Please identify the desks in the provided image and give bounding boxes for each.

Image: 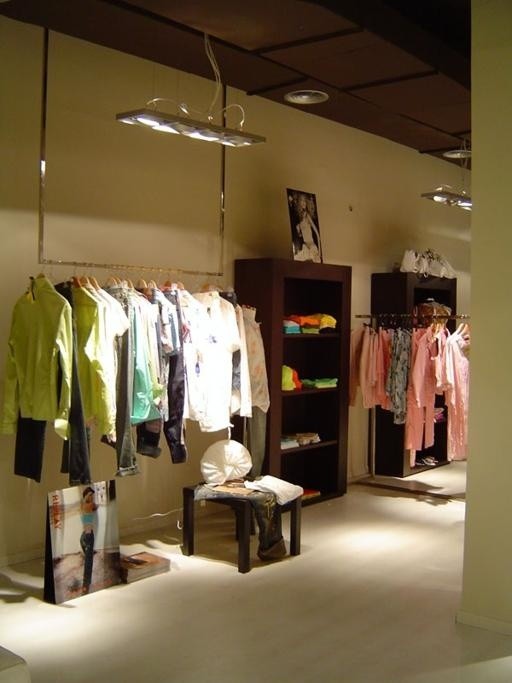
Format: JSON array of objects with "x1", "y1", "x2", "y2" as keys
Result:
[{"x1": 182, "y1": 478, "x2": 302, "y2": 572}]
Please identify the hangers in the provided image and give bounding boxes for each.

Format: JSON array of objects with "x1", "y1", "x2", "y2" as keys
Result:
[
  {"x1": 41, "y1": 261, "x2": 222, "y2": 294},
  {"x1": 366, "y1": 315, "x2": 464, "y2": 332}
]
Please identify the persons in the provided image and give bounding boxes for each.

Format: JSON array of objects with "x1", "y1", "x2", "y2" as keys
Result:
[
  {"x1": 79, "y1": 487, "x2": 99, "y2": 597},
  {"x1": 293, "y1": 194, "x2": 322, "y2": 264}
]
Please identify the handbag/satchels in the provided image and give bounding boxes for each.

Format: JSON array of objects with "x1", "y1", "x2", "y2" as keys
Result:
[
  {"x1": 400, "y1": 250, "x2": 430, "y2": 277},
  {"x1": 425, "y1": 250, "x2": 446, "y2": 278},
  {"x1": 439, "y1": 255, "x2": 457, "y2": 279}
]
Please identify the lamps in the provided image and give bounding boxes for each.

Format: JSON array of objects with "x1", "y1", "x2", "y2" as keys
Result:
[
  {"x1": 421, "y1": 140, "x2": 472, "y2": 212},
  {"x1": 117, "y1": 36, "x2": 266, "y2": 148}
]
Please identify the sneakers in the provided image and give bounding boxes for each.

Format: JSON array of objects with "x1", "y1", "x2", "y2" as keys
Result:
[{"x1": 415, "y1": 456, "x2": 439, "y2": 467}]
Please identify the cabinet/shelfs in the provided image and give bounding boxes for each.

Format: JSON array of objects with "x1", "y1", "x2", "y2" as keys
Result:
[
  {"x1": 230, "y1": 259, "x2": 351, "y2": 512},
  {"x1": 369, "y1": 273, "x2": 457, "y2": 478}
]
